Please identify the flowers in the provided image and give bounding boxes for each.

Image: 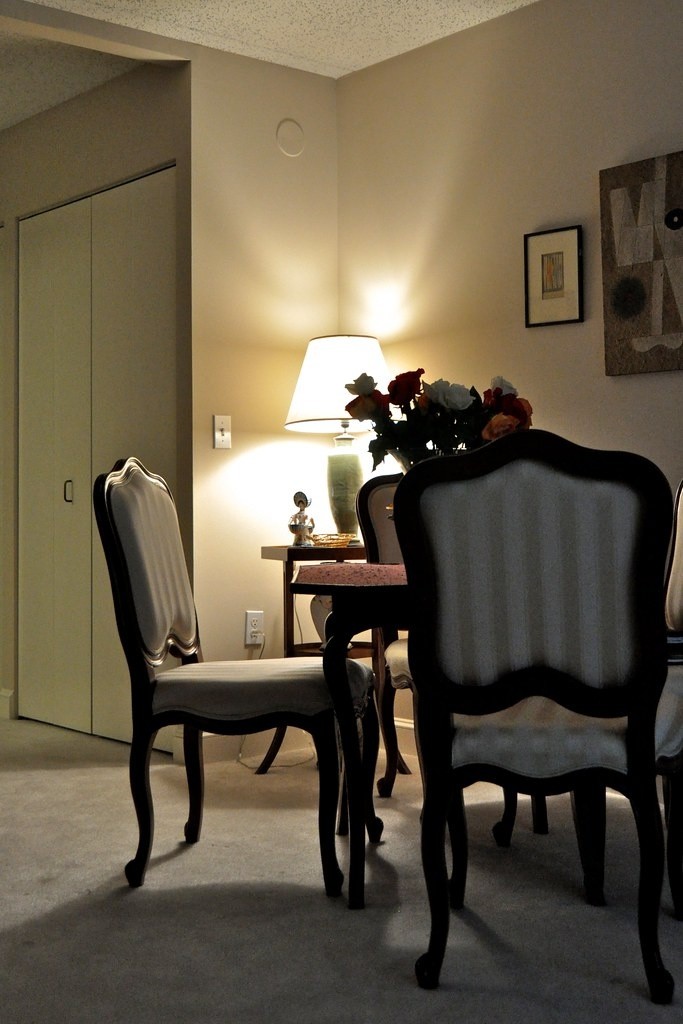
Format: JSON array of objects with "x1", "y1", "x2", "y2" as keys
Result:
[{"x1": 342, "y1": 368, "x2": 533, "y2": 473}]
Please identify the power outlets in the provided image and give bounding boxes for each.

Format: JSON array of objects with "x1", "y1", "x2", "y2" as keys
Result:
[{"x1": 246, "y1": 609, "x2": 266, "y2": 645}]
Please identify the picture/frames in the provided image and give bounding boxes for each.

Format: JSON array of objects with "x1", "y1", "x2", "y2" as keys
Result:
[{"x1": 524, "y1": 225, "x2": 584, "y2": 327}]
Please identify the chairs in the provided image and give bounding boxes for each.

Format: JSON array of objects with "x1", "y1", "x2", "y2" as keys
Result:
[
  {"x1": 95, "y1": 458, "x2": 384, "y2": 895},
  {"x1": 353, "y1": 473, "x2": 409, "y2": 795},
  {"x1": 393, "y1": 429, "x2": 676, "y2": 1006}
]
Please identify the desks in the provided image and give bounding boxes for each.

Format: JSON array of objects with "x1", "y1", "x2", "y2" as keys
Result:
[
  {"x1": 287, "y1": 561, "x2": 681, "y2": 909},
  {"x1": 253, "y1": 546, "x2": 413, "y2": 776}
]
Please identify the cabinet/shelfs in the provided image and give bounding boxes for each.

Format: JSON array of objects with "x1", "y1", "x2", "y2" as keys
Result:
[{"x1": 18, "y1": 161, "x2": 180, "y2": 754}]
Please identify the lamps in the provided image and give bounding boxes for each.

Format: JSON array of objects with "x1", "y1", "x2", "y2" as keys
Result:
[{"x1": 285, "y1": 334, "x2": 407, "y2": 543}]
